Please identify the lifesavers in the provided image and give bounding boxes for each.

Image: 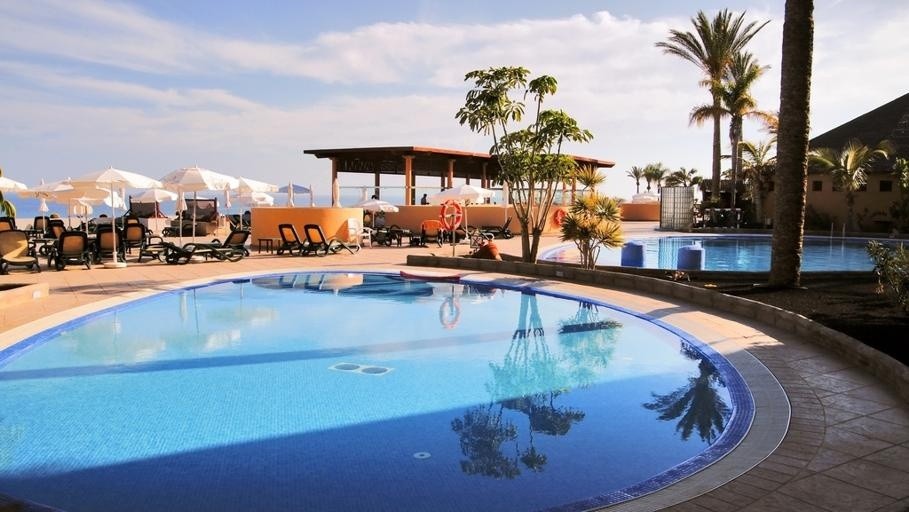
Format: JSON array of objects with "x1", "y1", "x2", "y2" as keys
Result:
[
  {"x1": 554, "y1": 208, "x2": 567, "y2": 226},
  {"x1": 440, "y1": 200, "x2": 462, "y2": 230},
  {"x1": 440, "y1": 301, "x2": 461, "y2": 328}
]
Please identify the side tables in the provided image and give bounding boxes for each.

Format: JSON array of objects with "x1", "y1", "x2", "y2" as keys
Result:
[{"x1": 259, "y1": 238, "x2": 281, "y2": 254}]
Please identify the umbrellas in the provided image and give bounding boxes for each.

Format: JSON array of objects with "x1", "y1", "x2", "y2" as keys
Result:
[
  {"x1": 353, "y1": 198, "x2": 399, "y2": 228},
  {"x1": 332, "y1": 178, "x2": 342, "y2": 209},
  {"x1": 361, "y1": 186, "x2": 369, "y2": 200}
]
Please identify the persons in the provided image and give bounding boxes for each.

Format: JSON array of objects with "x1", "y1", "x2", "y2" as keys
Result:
[
  {"x1": 421, "y1": 193, "x2": 430, "y2": 205},
  {"x1": 363, "y1": 211, "x2": 386, "y2": 246}
]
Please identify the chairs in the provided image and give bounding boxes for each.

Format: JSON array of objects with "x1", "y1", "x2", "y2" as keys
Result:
[
  {"x1": 278, "y1": 224, "x2": 310, "y2": 257},
  {"x1": 371, "y1": 217, "x2": 512, "y2": 247},
  {"x1": 306, "y1": 274, "x2": 324, "y2": 289},
  {"x1": 279, "y1": 273, "x2": 299, "y2": 287},
  {"x1": 298, "y1": 224, "x2": 361, "y2": 257},
  {"x1": 1, "y1": 215, "x2": 252, "y2": 275}
]
